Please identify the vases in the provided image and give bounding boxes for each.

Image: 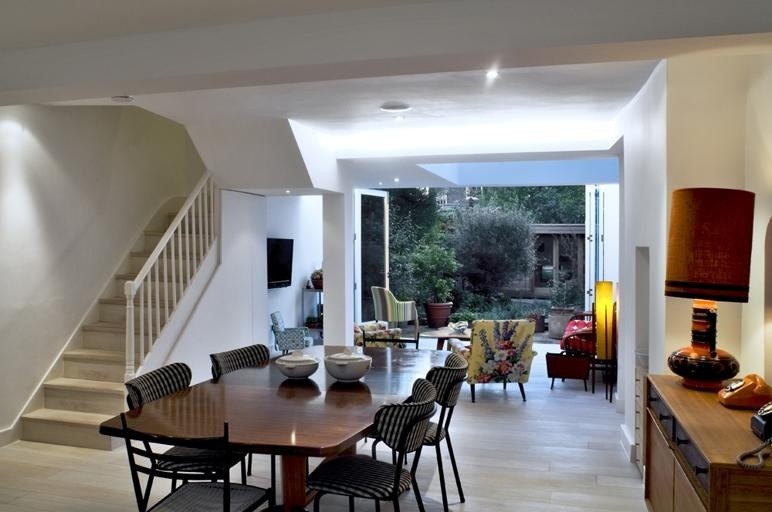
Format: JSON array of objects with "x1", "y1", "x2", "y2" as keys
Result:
[
  {"x1": 527, "y1": 314, "x2": 544, "y2": 332},
  {"x1": 313, "y1": 279, "x2": 322, "y2": 289}
]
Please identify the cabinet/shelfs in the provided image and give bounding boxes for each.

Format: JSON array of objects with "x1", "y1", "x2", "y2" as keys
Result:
[
  {"x1": 302, "y1": 288, "x2": 323, "y2": 339},
  {"x1": 641, "y1": 374, "x2": 772, "y2": 512}
]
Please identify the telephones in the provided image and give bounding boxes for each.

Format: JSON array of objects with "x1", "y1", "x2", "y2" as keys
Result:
[
  {"x1": 737, "y1": 400, "x2": 772, "y2": 470},
  {"x1": 717, "y1": 374, "x2": 772, "y2": 410}
]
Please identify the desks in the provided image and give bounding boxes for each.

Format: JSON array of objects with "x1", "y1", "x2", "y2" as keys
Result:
[{"x1": 99, "y1": 344, "x2": 454, "y2": 512}]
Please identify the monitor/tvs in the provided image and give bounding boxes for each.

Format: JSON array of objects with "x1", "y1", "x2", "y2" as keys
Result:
[{"x1": 267, "y1": 238, "x2": 293, "y2": 289}]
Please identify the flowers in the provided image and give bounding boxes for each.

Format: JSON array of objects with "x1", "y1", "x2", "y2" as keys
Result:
[{"x1": 311, "y1": 270, "x2": 323, "y2": 279}]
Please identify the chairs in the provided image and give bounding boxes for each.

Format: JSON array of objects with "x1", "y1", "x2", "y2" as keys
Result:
[
  {"x1": 307, "y1": 378, "x2": 438, "y2": 512},
  {"x1": 125, "y1": 362, "x2": 246, "y2": 508},
  {"x1": 371, "y1": 286, "x2": 419, "y2": 339},
  {"x1": 354, "y1": 320, "x2": 402, "y2": 348},
  {"x1": 209, "y1": 344, "x2": 309, "y2": 477},
  {"x1": 271, "y1": 311, "x2": 313, "y2": 354},
  {"x1": 120, "y1": 412, "x2": 274, "y2": 512},
  {"x1": 365, "y1": 352, "x2": 470, "y2": 512},
  {"x1": 446, "y1": 319, "x2": 538, "y2": 402}
]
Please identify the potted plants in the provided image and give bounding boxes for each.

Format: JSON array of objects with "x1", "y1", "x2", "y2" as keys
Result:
[
  {"x1": 407, "y1": 244, "x2": 464, "y2": 327},
  {"x1": 550, "y1": 280, "x2": 583, "y2": 314}
]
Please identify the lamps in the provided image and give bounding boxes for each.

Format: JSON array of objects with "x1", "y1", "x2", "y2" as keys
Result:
[
  {"x1": 595, "y1": 281, "x2": 613, "y2": 363},
  {"x1": 665, "y1": 188, "x2": 756, "y2": 392}
]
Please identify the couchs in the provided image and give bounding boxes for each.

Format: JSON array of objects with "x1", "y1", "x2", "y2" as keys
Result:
[{"x1": 560, "y1": 311, "x2": 613, "y2": 382}]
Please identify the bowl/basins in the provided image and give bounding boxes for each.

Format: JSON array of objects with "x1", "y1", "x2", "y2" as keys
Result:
[
  {"x1": 324, "y1": 351, "x2": 373, "y2": 383},
  {"x1": 275, "y1": 351, "x2": 321, "y2": 380}
]
